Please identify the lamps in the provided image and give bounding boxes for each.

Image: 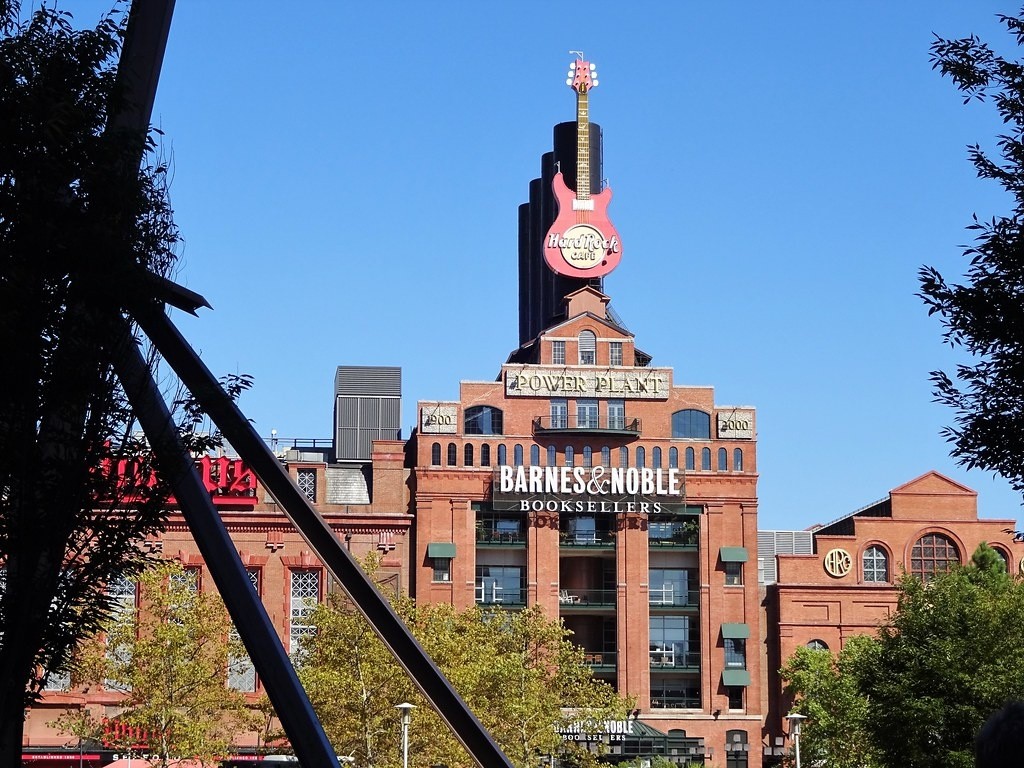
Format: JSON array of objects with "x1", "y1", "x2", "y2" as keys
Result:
[
  {"x1": 640, "y1": 367, "x2": 658, "y2": 392},
  {"x1": 722, "y1": 405, "x2": 742, "y2": 431},
  {"x1": 595, "y1": 366, "x2": 616, "y2": 391},
  {"x1": 553, "y1": 366, "x2": 572, "y2": 390},
  {"x1": 429, "y1": 401, "x2": 443, "y2": 418},
  {"x1": 514, "y1": 363, "x2": 529, "y2": 383}
]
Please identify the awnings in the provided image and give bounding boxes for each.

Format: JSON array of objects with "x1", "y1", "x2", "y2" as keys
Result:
[
  {"x1": 720, "y1": 547, "x2": 748, "y2": 562},
  {"x1": 722, "y1": 622, "x2": 748, "y2": 639},
  {"x1": 722, "y1": 669, "x2": 750, "y2": 686},
  {"x1": 428, "y1": 542, "x2": 455, "y2": 559}
]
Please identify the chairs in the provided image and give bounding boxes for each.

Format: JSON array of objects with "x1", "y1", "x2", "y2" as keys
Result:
[
  {"x1": 584, "y1": 654, "x2": 603, "y2": 664},
  {"x1": 559, "y1": 588, "x2": 580, "y2": 604}
]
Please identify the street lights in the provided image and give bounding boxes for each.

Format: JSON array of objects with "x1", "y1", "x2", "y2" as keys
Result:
[
  {"x1": 392, "y1": 701, "x2": 417, "y2": 768},
  {"x1": 786, "y1": 711, "x2": 808, "y2": 768}
]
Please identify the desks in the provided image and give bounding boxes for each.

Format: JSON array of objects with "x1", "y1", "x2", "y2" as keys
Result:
[{"x1": 569, "y1": 595, "x2": 578, "y2": 604}]
